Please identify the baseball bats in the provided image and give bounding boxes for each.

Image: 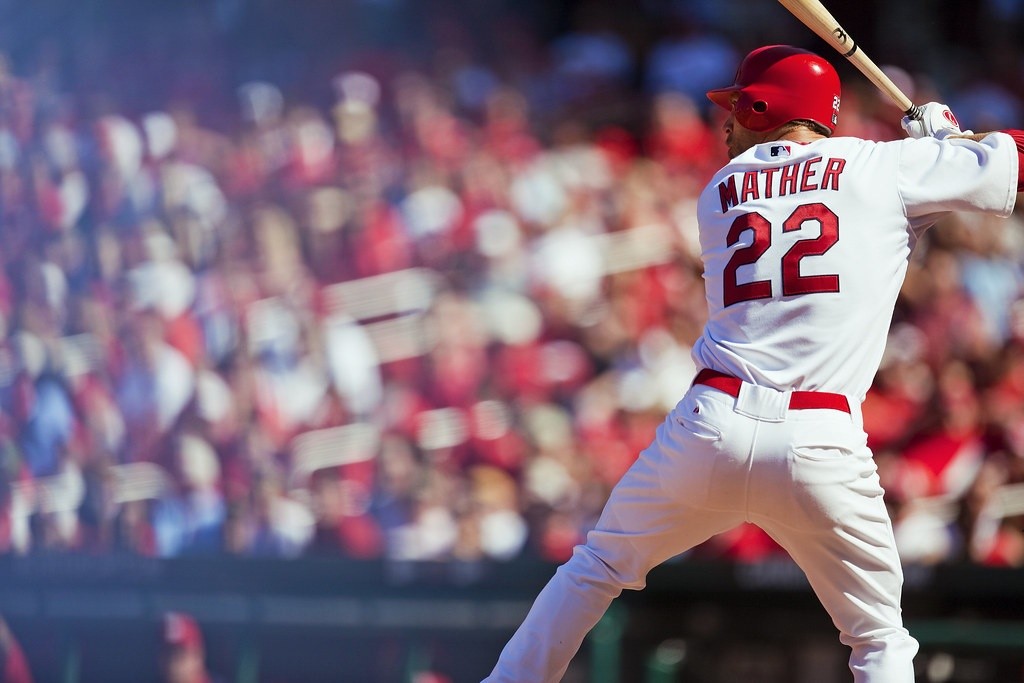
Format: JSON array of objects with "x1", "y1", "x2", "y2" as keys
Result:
[{"x1": 778, "y1": 1, "x2": 922, "y2": 120}]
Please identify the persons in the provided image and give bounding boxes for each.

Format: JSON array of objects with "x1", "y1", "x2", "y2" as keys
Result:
[
  {"x1": 468, "y1": 42, "x2": 1024, "y2": 683},
  {"x1": 156, "y1": 609, "x2": 212, "y2": 682},
  {"x1": 0, "y1": 1, "x2": 1024, "y2": 567}
]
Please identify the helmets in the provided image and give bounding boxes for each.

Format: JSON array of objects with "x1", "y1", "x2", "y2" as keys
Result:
[{"x1": 707, "y1": 45, "x2": 840, "y2": 132}]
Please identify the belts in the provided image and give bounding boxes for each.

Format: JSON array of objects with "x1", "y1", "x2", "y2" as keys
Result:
[{"x1": 689, "y1": 369, "x2": 850, "y2": 414}]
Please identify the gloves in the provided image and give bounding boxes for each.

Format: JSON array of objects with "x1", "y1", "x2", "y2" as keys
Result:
[{"x1": 900, "y1": 100, "x2": 973, "y2": 139}]
